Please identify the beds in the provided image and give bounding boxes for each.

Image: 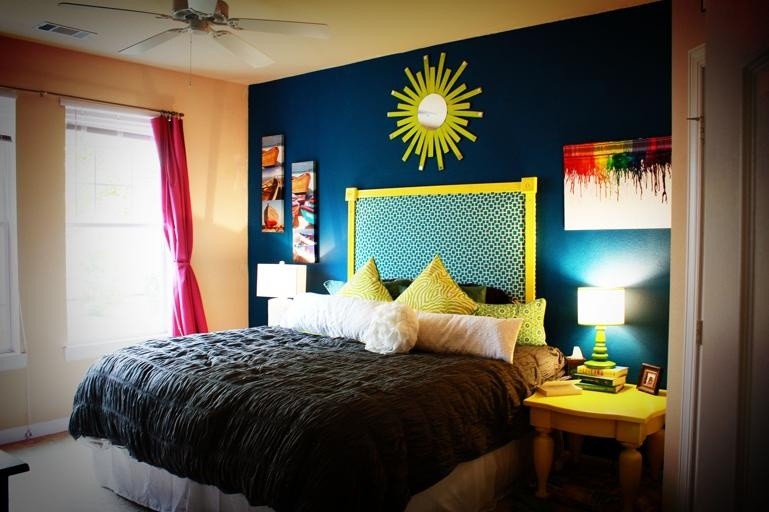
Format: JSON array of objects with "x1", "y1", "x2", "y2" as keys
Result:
[{"x1": 81, "y1": 326, "x2": 566, "y2": 508}]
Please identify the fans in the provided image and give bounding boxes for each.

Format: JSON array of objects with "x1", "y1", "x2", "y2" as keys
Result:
[{"x1": 57, "y1": 0, "x2": 332, "y2": 73}]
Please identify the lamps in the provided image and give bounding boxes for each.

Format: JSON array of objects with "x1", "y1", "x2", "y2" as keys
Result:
[
  {"x1": 255, "y1": 261, "x2": 307, "y2": 326},
  {"x1": 575, "y1": 285, "x2": 627, "y2": 368}
]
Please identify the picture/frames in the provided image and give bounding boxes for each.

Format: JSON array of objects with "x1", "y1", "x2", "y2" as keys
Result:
[{"x1": 636, "y1": 361, "x2": 662, "y2": 395}]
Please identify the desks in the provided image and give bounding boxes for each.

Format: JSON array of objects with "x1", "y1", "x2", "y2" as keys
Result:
[{"x1": 523, "y1": 377, "x2": 667, "y2": 512}]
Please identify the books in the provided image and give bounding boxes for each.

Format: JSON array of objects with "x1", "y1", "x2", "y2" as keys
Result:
[
  {"x1": 573, "y1": 363, "x2": 629, "y2": 393},
  {"x1": 535, "y1": 379, "x2": 583, "y2": 397}
]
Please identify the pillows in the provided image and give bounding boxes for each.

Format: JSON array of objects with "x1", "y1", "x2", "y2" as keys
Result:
[{"x1": 286, "y1": 254, "x2": 548, "y2": 367}]
